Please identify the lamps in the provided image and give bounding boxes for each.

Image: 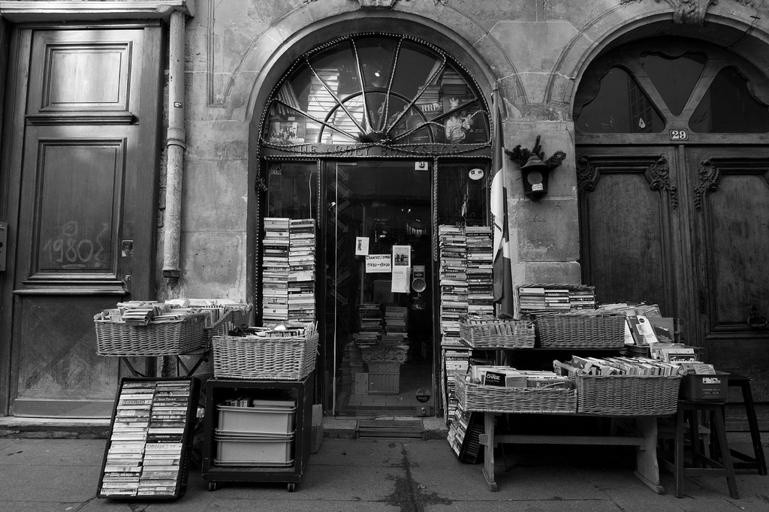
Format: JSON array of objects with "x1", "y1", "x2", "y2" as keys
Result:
[{"x1": 519, "y1": 151, "x2": 553, "y2": 202}]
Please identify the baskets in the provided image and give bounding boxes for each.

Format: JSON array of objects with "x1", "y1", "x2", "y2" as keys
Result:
[
  {"x1": 538, "y1": 312, "x2": 627, "y2": 349},
  {"x1": 212, "y1": 328, "x2": 319, "y2": 380},
  {"x1": 460, "y1": 317, "x2": 536, "y2": 349},
  {"x1": 577, "y1": 375, "x2": 681, "y2": 416},
  {"x1": 454, "y1": 374, "x2": 578, "y2": 411},
  {"x1": 94, "y1": 302, "x2": 253, "y2": 354}
]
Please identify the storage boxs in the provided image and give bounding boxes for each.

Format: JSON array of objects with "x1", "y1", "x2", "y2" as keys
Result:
[
  {"x1": 686, "y1": 368, "x2": 730, "y2": 399},
  {"x1": 212, "y1": 394, "x2": 297, "y2": 469}
]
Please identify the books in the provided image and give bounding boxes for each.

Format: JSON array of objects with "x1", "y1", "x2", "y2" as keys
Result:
[
  {"x1": 107, "y1": 298, "x2": 247, "y2": 328},
  {"x1": 458, "y1": 284, "x2": 659, "y2": 346},
  {"x1": 238, "y1": 217, "x2": 316, "y2": 340},
  {"x1": 471, "y1": 341, "x2": 716, "y2": 388},
  {"x1": 438, "y1": 224, "x2": 494, "y2": 423},
  {"x1": 353, "y1": 302, "x2": 408, "y2": 349},
  {"x1": 99, "y1": 381, "x2": 191, "y2": 495}
]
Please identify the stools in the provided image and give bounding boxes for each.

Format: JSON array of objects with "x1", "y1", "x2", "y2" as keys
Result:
[
  {"x1": 716, "y1": 372, "x2": 768, "y2": 476},
  {"x1": 675, "y1": 397, "x2": 742, "y2": 499}
]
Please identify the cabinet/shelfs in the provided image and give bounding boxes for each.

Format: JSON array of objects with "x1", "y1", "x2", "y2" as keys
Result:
[
  {"x1": 454, "y1": 337, "x2": 666, "y2": 495},
  {"x1": 196, "y1": 369, "x2": 318, "y2": 494}
]
